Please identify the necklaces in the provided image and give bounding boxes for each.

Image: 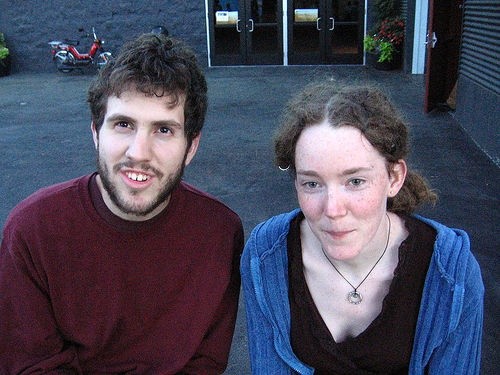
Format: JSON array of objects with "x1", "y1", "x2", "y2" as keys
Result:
[{"x1": 321, "y1": 213, "x2": 390, "y2": 303}]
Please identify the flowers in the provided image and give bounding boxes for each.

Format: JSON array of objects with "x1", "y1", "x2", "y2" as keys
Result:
[{"x1": 363, "y1": 16, "x2": 404, "y2": 63}]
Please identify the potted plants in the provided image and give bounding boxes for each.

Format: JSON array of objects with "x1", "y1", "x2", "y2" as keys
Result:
[{"x1": 0, "y1": 32, "x2": 11, "y2": 76}]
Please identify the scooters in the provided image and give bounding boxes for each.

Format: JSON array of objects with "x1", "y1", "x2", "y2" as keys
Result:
[{"x1": 47, "y1": 25, "x2": 115, "y2": 75}]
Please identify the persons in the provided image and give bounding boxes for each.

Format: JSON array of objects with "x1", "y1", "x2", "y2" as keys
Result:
[
  {"x1": 432, "y1": 0, "x2": 463, "y2": 113},
  {"x1": 0, "y1": 32, "x2": 244, "y2": 375},
  {"x1": 240, "y1": 74, "x2": 486, "y2": 375}
]
